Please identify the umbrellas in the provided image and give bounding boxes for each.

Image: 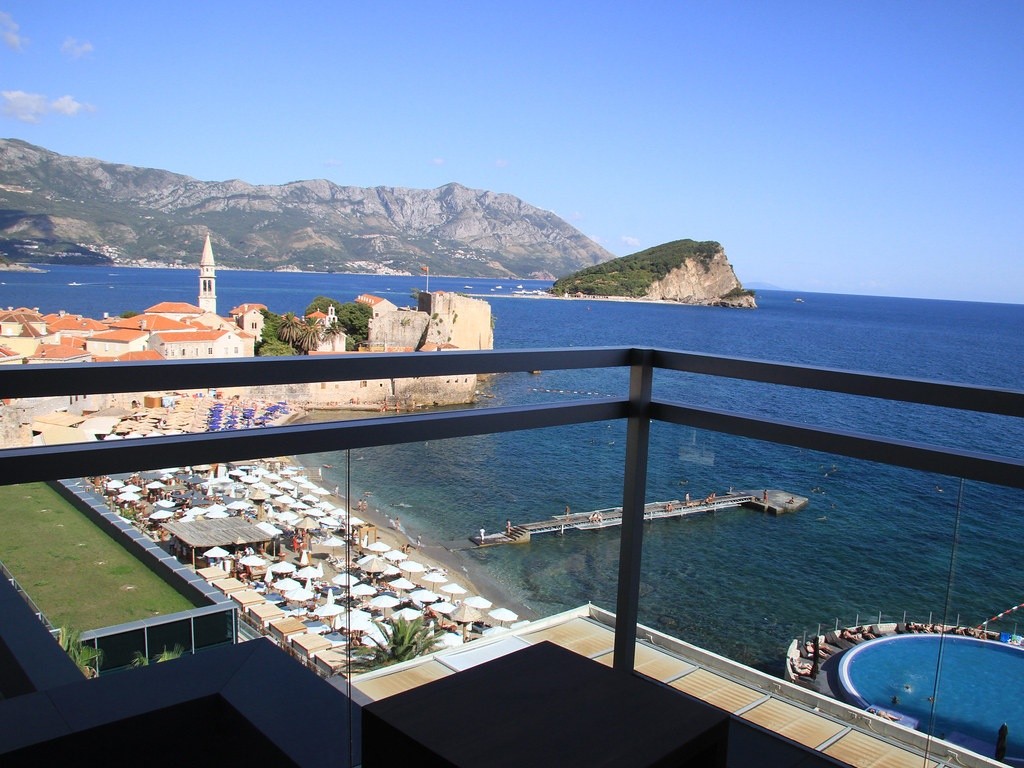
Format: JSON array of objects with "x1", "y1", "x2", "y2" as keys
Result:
[
  {"x1": 105, "y1": 457, "x2": 365, "y2": 536},
  {"x1": 204, "y1": 536, "x2": 517, "y2": 647},
  {"x1": 206, "y1": 400, "x2": 290, "y2": 432}
]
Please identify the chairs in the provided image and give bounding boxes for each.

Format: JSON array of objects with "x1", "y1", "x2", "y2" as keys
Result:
[
  {"x1": 897, "y1": 623, "x2": 995, "y2": 641},
  {"x1": 798, "y1": 621, "x2": 887, "y2": 663}
]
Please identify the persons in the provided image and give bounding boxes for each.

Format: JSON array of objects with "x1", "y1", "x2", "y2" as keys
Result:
[
  {"x1": 505, "y1": 518, "x2": 511, "y2": 536},
  {"x1": 906, "y1": 622, "x2": 943, "y2": 634},
  {"x1": 664, "y1": 501, "x2": 674, "y2": 512},
  {"x1": 704, "y1": 492, "x2": 716, "y2": 506},
  {"x1": 955, "y1": 626, "x2": 987, "y2": 640},
  {"x1": 565, "y1": 506, "x2": 570, "y2": 516},
  {"x1": 588, "y1": 511, "x2": 603, "y2": 523},
  {"x1": 480, "y1": 526, "x2": 485, "y2": 543},
  {"x1": 843, "y1": 630, "x2": 858, "y2": 640},
  {"x1": 861, "y1": 627, "x2": 876, "y2": 639},
  {"x1": 790, "y1": 658, "x2": 812, "y2": 676},
  {"x1": 763, "y1": 489, "x2": 768, "y2": 504},
  {"x1": 806, "y1": 639, "x2": 834, "y2": 660},
  {"x1": 790, "y1": 496, "x2": 794, "y2": 504}
]
